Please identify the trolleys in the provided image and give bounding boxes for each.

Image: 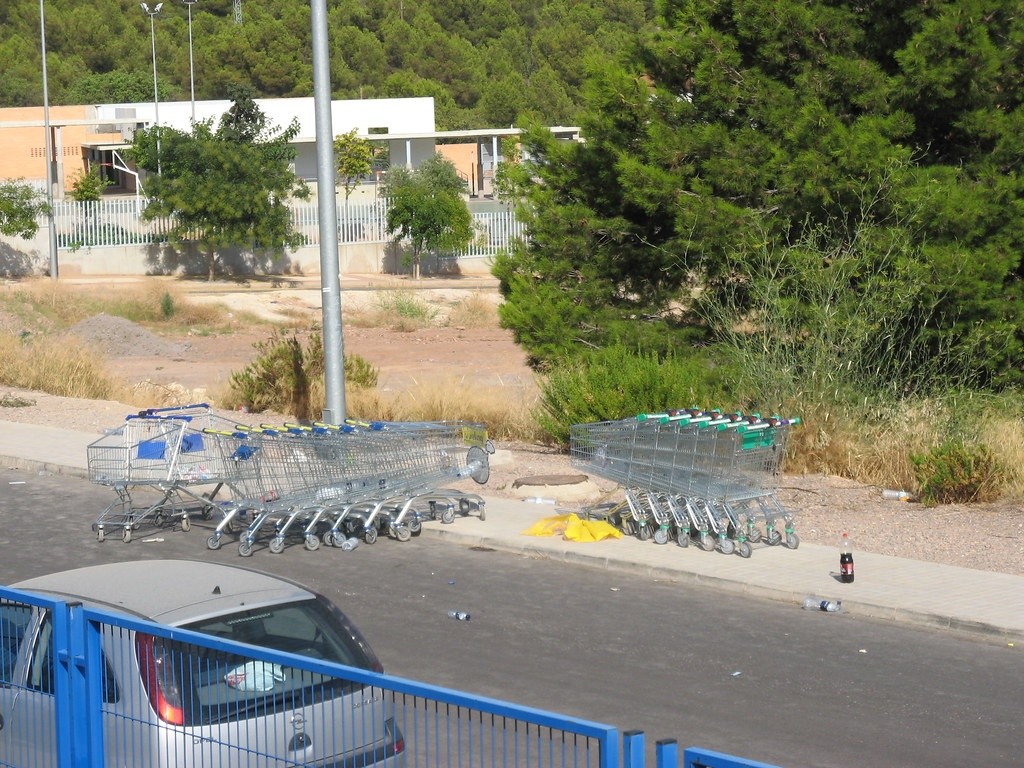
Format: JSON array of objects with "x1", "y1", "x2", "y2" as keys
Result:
[
  {"x1": 86, "y1": 414, "x2": 192, "y2": 542},
  {"x1": 139, "y1": 402, "x2": 495, "y2": 557},
  {"x1": 568, "y1": 405, "x2": 802, "y2": 561}
]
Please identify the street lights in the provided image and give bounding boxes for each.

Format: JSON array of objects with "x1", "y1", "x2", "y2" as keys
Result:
[{"x1": 140, "y1": 1, "x2": 164, "y2": 193}]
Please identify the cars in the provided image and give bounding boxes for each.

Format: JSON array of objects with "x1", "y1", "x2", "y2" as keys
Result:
[{"x1": 0, "y1": 557, "x2": 410, "y2": 768}]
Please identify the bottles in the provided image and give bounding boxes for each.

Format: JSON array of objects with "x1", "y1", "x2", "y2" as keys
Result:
[
  {"x1": 882, "y1": 489, "x2": 913, "y2": 501},
  {"x1": 458, "y1": 461, "x2": 482, "y2": 477},
  {"x1": 259, "y1": 489, "x2": 278, "y2": 502},
  {"x1": 521, "y1": 498, "x2": 557, "y2": 506},
  {"x1": 899, "y1": 492, "x2": 907, "y2": 514},
  {"x1": 447, "y1": 610, "x2": 470, "y2": 622},
  {"x1": 802, "y1": 598, "x2": 841, "y2": 612},
  {"x1": 316, "y1": 478, "x2": 387, "y2": 500},
  {"x1": 840, "y1": 533, "x2": 855, "y2": 582}
]
